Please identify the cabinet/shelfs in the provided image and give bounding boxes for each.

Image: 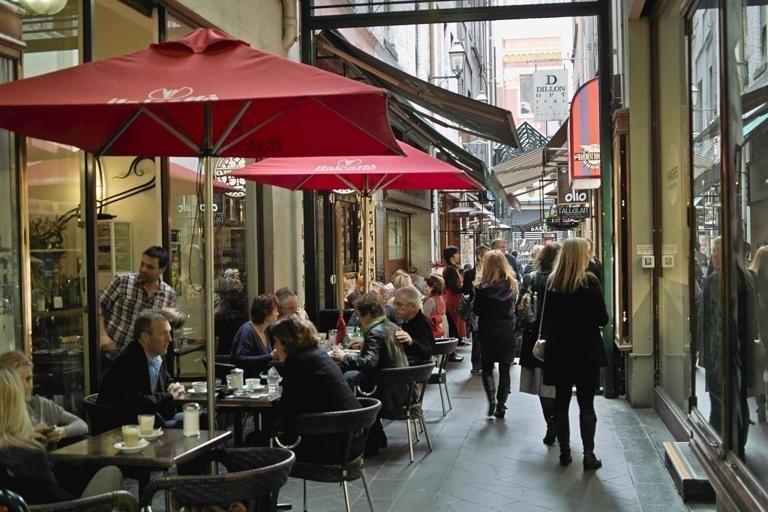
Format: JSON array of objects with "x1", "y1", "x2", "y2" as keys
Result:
[
  {"x1": 29, "y1": 249, "x2": 82, "y2": 318},
  {"x1": 97, "y1": 221, "x2": 131, "y2": 273}
]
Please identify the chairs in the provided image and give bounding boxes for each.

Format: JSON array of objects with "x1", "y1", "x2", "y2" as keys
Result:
[
  {"x1": 415, "y1": 337, "x2": 459, "y2": 416},
  {"x1": 83, "y1": 392, "x2": 122, "y2": 435},
  {"x1": 318, "y1": 310, "x2": 354, "y2": 338},
  {"x1": 270, "y1": 397, "x2": 382, "y2": 512},
  {"x1": 140, "y1": 448, "x2": 296, "y2": 511},
  {"x1": 429, "y1": 40, "x2": 466, "y2": 80},
  {"x1": 356, "y1": 360, "x2": 434, "y2": 462},
  {"x1": 0, "y1": 486, "x2": 137, "y2": 511},
  {"x1": 203, "y1": 353, "x2": 236, "y2": 382}
]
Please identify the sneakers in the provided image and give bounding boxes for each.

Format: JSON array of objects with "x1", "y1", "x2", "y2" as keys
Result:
[
  {"x1": 489, "y1": 399, "x2": 505, "y2": 418},
  {"x1": 448, "y1": 352, "x2": 464, "y2": 361},
  {"x1": 543, "y1": 415, "x2": 557, "y2": 444}
]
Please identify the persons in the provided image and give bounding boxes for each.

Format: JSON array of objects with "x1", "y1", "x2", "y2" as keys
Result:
[
  {"x1": 91, "y1": 310, "x2": 217, "y2": 432},
  {"x1": 230, "y1": 293, "x2": 281, "y2": 386},
  {"x1": 694, "y1": 235, "x2": 768, "y2": 461},
  {"x1": 255, "y1": 313, "x2": 364, "y2": 465},
  {"x1": 1, "y1": 349, "x2": 89, "y2": 492},
  {"x1": 331, "y1": 298, "x2": 408, "y2": 455},
  {"x1": 176, "y1": 269, "x2": 249, "y2": 355},
  {"x1": 344, "y1": 246, "x2": 470, "y2": 365},
  {"x1": 95, "y1": 246, "x2": 187, "y2": 371},
  {"x1": 462, "y1": 236, "x2": 610, "y2": 472},
  {"x1": 2, "y1": 367, "x2": 124, "y2": 510},
  {"x1": 272, "y1": 287, "x2": 310, "y2": 330}
]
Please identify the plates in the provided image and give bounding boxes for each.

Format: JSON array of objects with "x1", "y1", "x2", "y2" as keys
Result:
[
  {"x1": 138, "y1": 431, "x2": 164, "y2": 440},
  {"x1": 188, "y1": 388, "x2": 206, "y2": 394},
  {"x1": 114, "y1": 442, "x2": 150, "y2": 453}
]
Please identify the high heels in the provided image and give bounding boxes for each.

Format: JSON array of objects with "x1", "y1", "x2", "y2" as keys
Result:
[
  {"x1": 560, "y1": 451, "x2": 572, "y2": 465},
  {"x1": 584, "y1": 451, "x2": 602, "y2": 470}
]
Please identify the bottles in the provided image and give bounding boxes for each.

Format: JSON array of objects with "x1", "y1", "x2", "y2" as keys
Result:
[
  {"x1": 336, "y1": 311, "x2": 346, "y2": 342},
  {"x1": 36, "y1": 293, "x2": 45, "y2": 312},
  {"x1": 51, "y1": 271, "x2": 64, "y2": 310}
]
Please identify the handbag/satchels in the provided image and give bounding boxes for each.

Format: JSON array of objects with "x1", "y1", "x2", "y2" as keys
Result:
[
  {"x1": 532, "y1": 339, "x2": 547, "y2": 363},
  {"x1": 518, "y1": 271, "x2": 538, "y2": 323}
]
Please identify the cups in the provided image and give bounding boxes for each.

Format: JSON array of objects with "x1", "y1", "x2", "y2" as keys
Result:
[
  {"x1": 346, "y1": 326, "x2": 355, "y2": 338},
  {"x1": 182, "y1": 403, "x2": 200, "y2": 437},
  {"x1": 245, "y1": 377, "x2": 261, "y2": 387},
  {"x1": 267, "y1": 375, "x2": 279, "y2": 389},
  {"x1": 122, "y1": 425, "x2": 138, "y2": 447},
  {"x1": 329, "y1": 329, "x2": 337, "y2": 339},
  {"x1": 138, "y1": 414, "x2": 156, "y2": 434},
  {"x1": 192, "y1": 382, "x2": 206, "y2": 392},
  {"x1": 230, "y1": 368, "x2": 244, "y2": 387}
]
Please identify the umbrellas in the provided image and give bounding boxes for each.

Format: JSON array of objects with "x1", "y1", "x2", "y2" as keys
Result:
[
  {"x1": 0, "y1": 27, "x2": 408, "y2": 476},
  {"x1": 226, "y1": 138, "x2": 486, "y2": 296}
]
